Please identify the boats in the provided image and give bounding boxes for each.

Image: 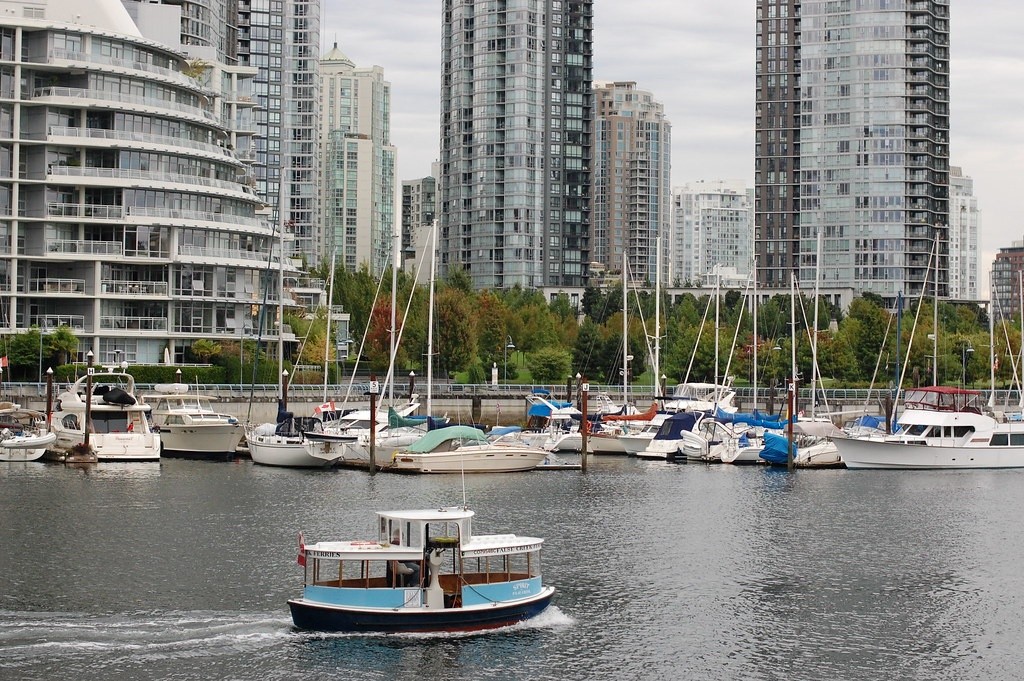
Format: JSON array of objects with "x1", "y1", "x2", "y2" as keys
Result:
[
  {"x1": 137, "y1": 382, "x2": 245, "y2": 460},
  {"x1": 0, "y1": 401, "x2": 57, "y2": 462},
  {"x1": 40, "y1": 361, "x2": 162, "y2": 463},
  {"x1": 286, "y1": 506, "x2": 557, "y2": 636}
]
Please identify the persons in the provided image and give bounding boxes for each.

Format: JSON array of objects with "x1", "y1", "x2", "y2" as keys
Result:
[
  {"x1": 404, "y1": 561, "x2": 421, "y2": 587},
  {"x1": 386, "y1": 529, "x2": 404, "y2": 588}
]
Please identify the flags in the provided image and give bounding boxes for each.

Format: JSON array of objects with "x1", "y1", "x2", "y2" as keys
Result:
[
  {"x1": 127, "y1": 422, "x2": 133, "y2": 431},
  {"x1": 297, "y1": 531, "x2": 307, "y2": 567},
  {"x1": 0, "y1": 356, "x2": 8, "y2": 367},
  {"x1": 315, "y1": 401, "x2": 336, "y2": 414}
]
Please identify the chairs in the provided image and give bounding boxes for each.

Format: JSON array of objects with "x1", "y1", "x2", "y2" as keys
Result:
[{"x1": 390, "y1": 559, "x2": 414, "y2": 588}]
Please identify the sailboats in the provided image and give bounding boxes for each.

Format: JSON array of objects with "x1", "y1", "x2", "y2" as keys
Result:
[{"x1": 237, "y1": 163, "x2": 1024, "y2": 470}]
